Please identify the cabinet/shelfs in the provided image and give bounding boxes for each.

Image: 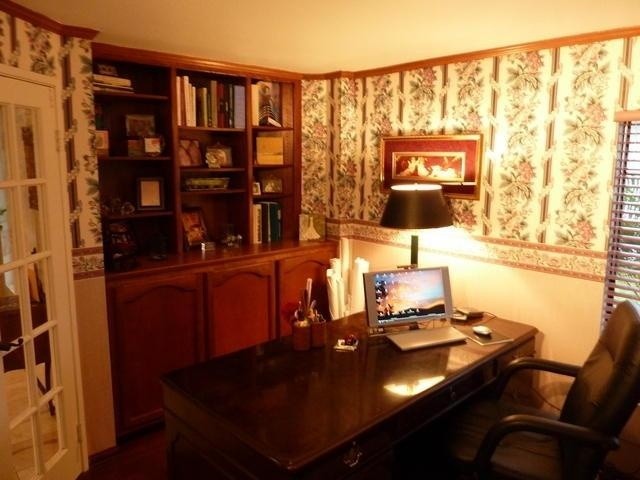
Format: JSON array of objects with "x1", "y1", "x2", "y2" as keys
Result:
[
  {"x1": 105, "y1": 236, "x2": 337, "y2": 439},
  {"x1": 91, "y1": 40, "x2": 303, "y2": 277}
]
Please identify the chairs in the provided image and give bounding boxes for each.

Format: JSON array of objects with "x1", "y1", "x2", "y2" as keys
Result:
[{"x1": 440, "y1": 298, "x2": 640, "y2": 479}]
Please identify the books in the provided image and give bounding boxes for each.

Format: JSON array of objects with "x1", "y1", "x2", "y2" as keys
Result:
[
  {"x1": 253, "y1": 201, "x2": 283, "y2": 244},
  {"x1": 461, "y1": 327, "x2": 514, "y2": 346},
  {"x1": 92, "y1": 74, "x2": 133, "y2": 93},
  {"x1": 251, "y1": 84, "x2": 259, "y2": 127},
  {"x1": 176, "y1": 75, "x2": 245, "y2": 128}
]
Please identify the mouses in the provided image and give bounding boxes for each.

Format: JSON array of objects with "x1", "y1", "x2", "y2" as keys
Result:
[{"x1": 471, "y1": 324, "x2": 492, "y2": 336}]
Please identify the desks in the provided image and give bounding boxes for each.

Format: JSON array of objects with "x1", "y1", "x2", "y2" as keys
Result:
[{"x1": 159, "y1": 308, "x2": 533, "y2": 480}]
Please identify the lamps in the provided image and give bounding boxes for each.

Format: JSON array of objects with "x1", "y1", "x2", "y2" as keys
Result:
[{"x1": 379, "y1": 184, "x2": 454, "y2": 269}]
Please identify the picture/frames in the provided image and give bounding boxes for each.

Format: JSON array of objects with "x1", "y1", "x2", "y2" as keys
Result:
[{"x1": 378, "y1": 134, "x2": 482, "y2": 200}]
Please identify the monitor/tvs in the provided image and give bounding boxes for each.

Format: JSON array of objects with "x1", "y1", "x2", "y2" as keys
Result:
[{"x1": 363, "y1": 265, "x2": 452, "y2": 329}]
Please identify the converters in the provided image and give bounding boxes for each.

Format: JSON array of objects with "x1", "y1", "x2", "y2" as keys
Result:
[{"x1": 456, "y1": 305, "x2": 484, "y2": 317}]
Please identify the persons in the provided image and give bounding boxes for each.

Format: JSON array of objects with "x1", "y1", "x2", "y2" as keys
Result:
[{"x1": 258, "y1": 86, "x2": 276, "y2": 126}]
